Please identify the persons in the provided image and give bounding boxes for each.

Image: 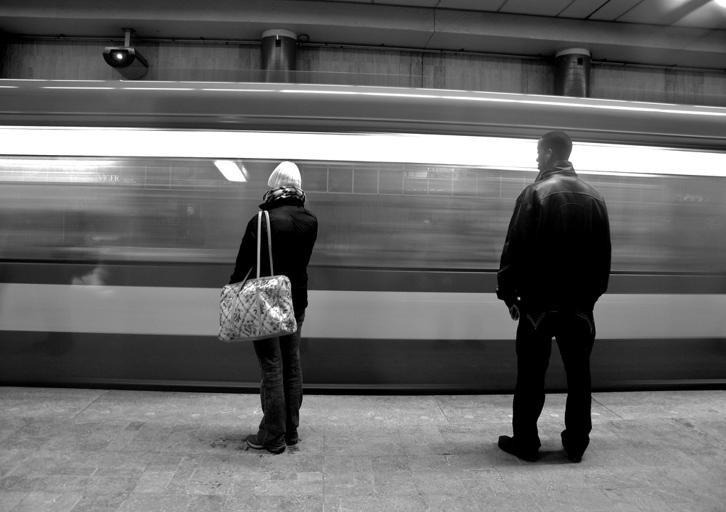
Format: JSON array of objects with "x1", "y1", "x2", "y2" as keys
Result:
[
  {"x1": 229, "y1": 161, "x2": 318, "y2": 454},
  {"x1": 496, "y1": 131, "x2": 611, "y2": 462}
]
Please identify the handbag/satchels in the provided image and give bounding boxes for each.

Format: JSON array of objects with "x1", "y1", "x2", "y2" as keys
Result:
[{"x1": 217, "y1": 275, "x2": 298, "y2": 344}]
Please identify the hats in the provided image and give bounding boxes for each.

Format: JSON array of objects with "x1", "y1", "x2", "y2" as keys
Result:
[{"x1": 267, "y1": 161, "x2": 301, "y2": 190}]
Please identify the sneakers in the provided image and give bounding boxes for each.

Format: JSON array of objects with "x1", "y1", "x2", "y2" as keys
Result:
[
  {"x1": 561, "y1": 429, "x2": 582, "y2": 463},
  {"x1": 246, "y1": 432, "x2": 298, "y2": 454},
  {"x1": 498, "y1": 435, "x2": 540, "y2": 462}
]
